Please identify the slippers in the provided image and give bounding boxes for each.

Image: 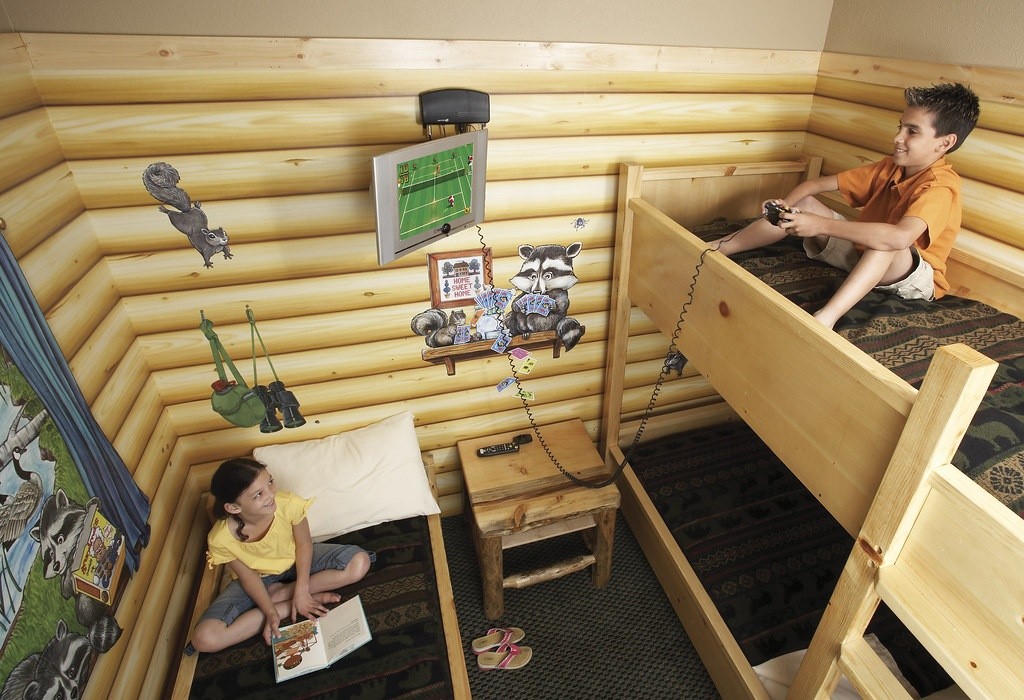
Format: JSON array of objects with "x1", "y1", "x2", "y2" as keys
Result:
[
  {"x1": 476, "y1": 642, "x2": 532, "y2": 671},
  {"x1": 471, "y1": 626, "x2": 525, "y2": 654}
]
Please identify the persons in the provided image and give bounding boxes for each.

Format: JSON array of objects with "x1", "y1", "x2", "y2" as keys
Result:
[
  {"x1": 709, "y1": 81, "x2": 980, "y2": 330},
  {"x1": 182, "y1": 459, "x2": 369, "y2": 657}
]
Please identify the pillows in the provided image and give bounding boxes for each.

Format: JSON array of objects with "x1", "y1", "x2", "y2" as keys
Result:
[{"x1": 253, "y1": 410, "x2": 441, "y2": 544}]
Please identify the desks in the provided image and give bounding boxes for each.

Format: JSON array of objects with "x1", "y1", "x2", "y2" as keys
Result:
[{"x1": 457, "y1": 418, "x2": 621, "y2": 621}]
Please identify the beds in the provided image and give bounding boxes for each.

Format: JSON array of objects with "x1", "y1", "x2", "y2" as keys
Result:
[
  {"x1": 598, "y1": 153, "x2": 1024, "y2": 700},
  {"x1": 170, "y1": 453, "x2": 473, "y2": 700}
]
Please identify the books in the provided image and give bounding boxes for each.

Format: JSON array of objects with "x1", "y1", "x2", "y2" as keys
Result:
[{"x1": 274, "y1": 594, "x2": 371, "y2": 682}]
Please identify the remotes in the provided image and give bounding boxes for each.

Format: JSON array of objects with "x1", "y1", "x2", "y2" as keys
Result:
[{"x1": 477, "y1": 443, "x2": 520, "y2": 457}]
